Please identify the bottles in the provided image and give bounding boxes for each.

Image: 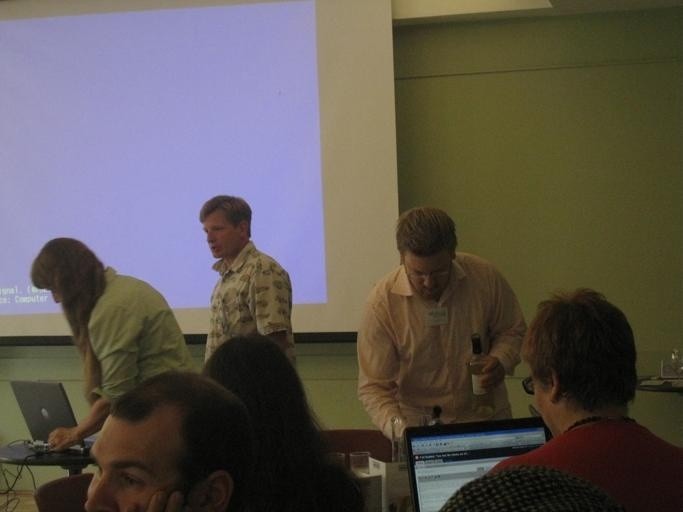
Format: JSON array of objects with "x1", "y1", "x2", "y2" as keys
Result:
[
  {"x1": 465, "y1": 331, "x2": 492, "y2": 400},
  {"x1": 429, "y1": 403, "x2": 444, "y2": 426},
  {"x1": 660, "y1": 346, "x2": 682, "y2": 381}
]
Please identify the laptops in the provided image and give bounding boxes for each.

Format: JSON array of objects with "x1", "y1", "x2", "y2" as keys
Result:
[
  {"x1": 9, "y1": 380, "x2": 95, "y2": 456},
  {"x1": 403, "y1": 416, "x2": 553, "y2": 512}
]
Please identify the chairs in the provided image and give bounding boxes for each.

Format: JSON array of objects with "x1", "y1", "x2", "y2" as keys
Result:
[
  {"x1": 32, "y1": 470, "x2": 96, "y2": 512},
  {"x1": 318, "y1": 428, "x2": 393, "y2": 462}
]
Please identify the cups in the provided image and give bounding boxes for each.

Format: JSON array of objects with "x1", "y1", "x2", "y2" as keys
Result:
[
  {"x1": 392, "y1": 413, "x2": 432, "y2": 469},
  {"x1": 349, "y1": 451, "x2": 370, "y2": 474}
]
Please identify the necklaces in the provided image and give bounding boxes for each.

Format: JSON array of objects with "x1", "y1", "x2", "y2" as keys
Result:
[{"x1": 563, "y1": 415, "x2": 637, "y2": 434}]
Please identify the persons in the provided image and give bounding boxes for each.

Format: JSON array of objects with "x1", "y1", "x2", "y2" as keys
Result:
[
  {"x1": 197, "y1": 194, "x2": 296, "y2": 370},
  {"x1": 81, "y1": 370, "x2": 251, "y2": 512},
  {"x1": 355, "y1": 207, "x2": 528, "y2": 448},
  {"x1": 437, "y1": 464, "x2": 623, "y2": 512},
  {"x1": 30, "y1": 238, "x2": 196, "y2": 453},
  {"x1": 203, "y1": 336, "x2": 365, "y2": 512},
  {"x1": 486, "y1": 285, "x2": 682, "y2": 511}
]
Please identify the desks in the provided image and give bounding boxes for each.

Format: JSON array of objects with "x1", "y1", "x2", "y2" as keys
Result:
[{"x1": 0, "y1": 435, "x2": 98, "y2": 477}]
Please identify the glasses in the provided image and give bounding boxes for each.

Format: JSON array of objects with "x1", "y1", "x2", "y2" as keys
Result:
[
  {"x1": 522, "y1": 376, "x2": 536, "y2": 395},
  {"x1": 403, "y1": 259, "x2": 451, "y2": 281}
]
